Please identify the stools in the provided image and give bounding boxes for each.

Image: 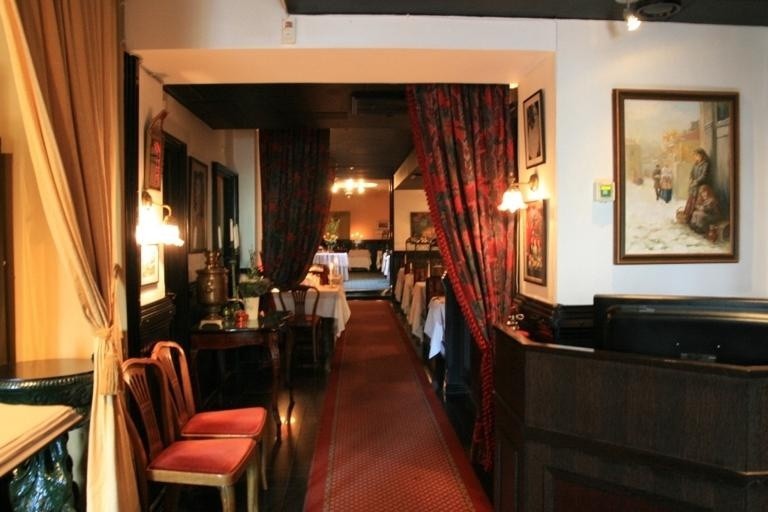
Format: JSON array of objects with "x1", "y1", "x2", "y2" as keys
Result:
[{"x1": 709, "y1": 220, "x2": 729, "y2": 241}]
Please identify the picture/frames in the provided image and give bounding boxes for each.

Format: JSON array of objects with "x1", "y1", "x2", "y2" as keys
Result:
[
  {"x1": 139, "y1": 246, "x2": 159, "y2": 286},
  {"x1": 523, "y1": 88, "x2": 547, "y2": 169},
  {"x1": 522, "y1": 198, "x2": 549, "y2": 286},
  {"x1": 611, "y1": 87, "x2": 744, "y2": 265}
]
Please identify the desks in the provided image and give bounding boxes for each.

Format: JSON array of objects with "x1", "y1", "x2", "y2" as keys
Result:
[
  {"x1": 348, "y1": 249, "x2": 371, "y2": 271},
  {"x1": 190, "y1": 311, "x2": 296, "y2": 425}
]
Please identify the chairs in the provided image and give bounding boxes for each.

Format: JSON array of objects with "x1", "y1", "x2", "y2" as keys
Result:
[
  {"x1": 270, "y1": 253, "x2": 348, "y2": 363},
  {"x1": 116, "y1": 341, "x2": 270, "y2": 511}
]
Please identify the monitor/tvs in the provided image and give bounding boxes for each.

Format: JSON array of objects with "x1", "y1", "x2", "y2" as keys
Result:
[{"x1": 592, "y1": 295, "x2": 768, "y2": 368}]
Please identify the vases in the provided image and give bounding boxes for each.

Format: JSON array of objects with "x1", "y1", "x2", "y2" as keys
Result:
[{"x1": 327, "y1": 245, "x2": 334, "y2": 253}]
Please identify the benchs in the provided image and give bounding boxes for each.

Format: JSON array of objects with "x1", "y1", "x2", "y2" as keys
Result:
[{"x1": 381, "y1": 248, "x2": 478, "y2": 403}]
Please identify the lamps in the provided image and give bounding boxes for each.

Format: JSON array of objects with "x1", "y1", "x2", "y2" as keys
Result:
[
  {"x1": 496, "y1": 172, "x2": 539, "y2": 213},
  {"x1": 133, "y1": 191, "x2": 185, "y2": 249}
]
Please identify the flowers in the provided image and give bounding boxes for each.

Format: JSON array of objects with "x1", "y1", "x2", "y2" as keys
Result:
[{"x1": 323, "y1": 218, "x2": 340, "y2": 246}]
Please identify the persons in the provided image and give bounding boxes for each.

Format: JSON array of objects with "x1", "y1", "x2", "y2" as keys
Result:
[
  {"x1": 652, "y1": 165, "x2": 662, "y2": 200},
  {"x1": 689, "y1": 185, "x2": 722, "y2": 234},
  {"x1": 684, "y1": 147, "x2": 710, "y2": 223},
  {"x1": 659, "y1": 165, "x2": 673, "y2": 203}
]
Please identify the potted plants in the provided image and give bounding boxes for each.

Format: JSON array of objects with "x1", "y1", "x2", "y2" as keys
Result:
[{"x1": 238, "y1": 278, "x2": 270, "y2": 321}]
